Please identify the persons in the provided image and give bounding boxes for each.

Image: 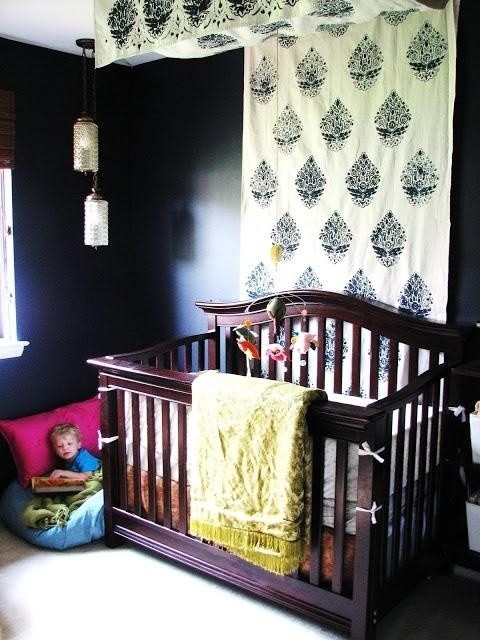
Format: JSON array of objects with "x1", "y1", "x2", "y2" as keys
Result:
[{"x1": 46, "y1": 423, "x2": 100, "y2": 481}]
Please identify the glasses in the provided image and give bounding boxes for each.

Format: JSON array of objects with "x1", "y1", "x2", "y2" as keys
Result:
[{"x1": 72, "y1": 39, "x2": 109, "y2": 249}]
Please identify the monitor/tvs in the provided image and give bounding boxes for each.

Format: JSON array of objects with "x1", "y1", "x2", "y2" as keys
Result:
[{"x1": 31, "y1": 476, "x2": 86, "y2": 494}]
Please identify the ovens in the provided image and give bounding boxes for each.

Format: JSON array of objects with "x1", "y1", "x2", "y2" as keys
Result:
[{"x1": 86, "y1": 290, "x2": 470, "y2": 640}]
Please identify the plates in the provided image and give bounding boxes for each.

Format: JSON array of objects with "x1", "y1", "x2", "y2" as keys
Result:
[{"x1": 438, "y1": 360, "x2": 480, "y2": 576}]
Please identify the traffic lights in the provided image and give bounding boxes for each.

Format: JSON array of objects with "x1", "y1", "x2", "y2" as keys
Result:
[{"x1": 0, "y1": 395, "x2": 101, "y2": 489}]
